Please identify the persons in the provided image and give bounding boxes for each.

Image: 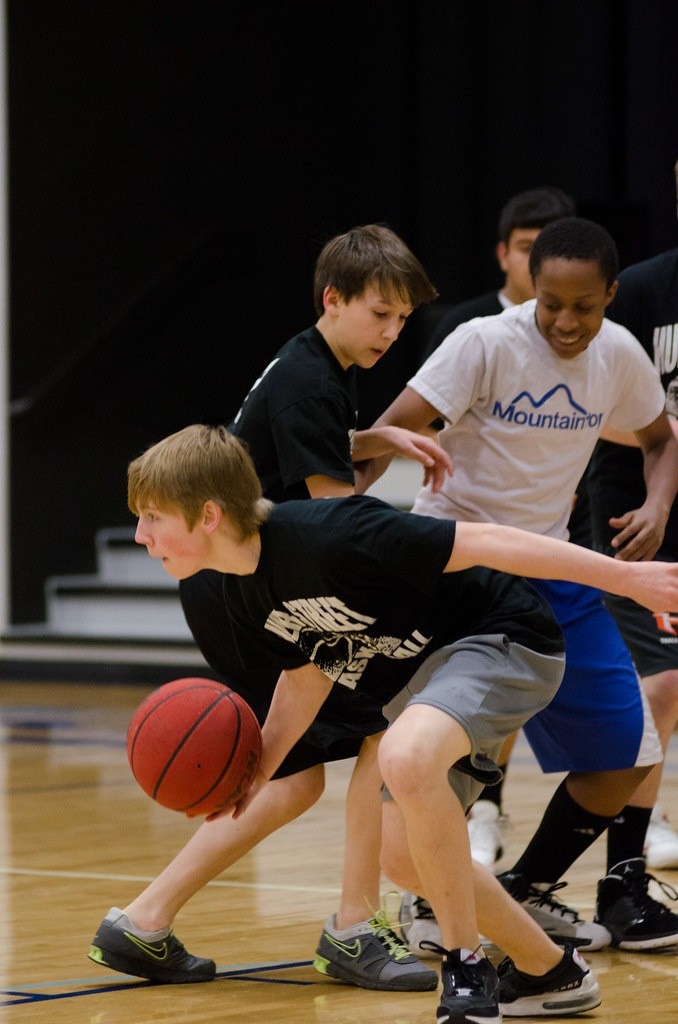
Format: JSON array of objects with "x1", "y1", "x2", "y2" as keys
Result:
[
  {"x1": 585, "y1": 250, "x2": 678, "y2": 949},
  {"x1": 468, "y1": 193, "x2": 678, "y2": 871},
  {"x1": 127, "y1": 424, "x2": 678, "y2": 1024},
  {"x1": 353, "y1": 216, "x2": 678, "y2": 959},
  {"x1": 86, "y1": 223, "x2": 455, "y2": 991}
]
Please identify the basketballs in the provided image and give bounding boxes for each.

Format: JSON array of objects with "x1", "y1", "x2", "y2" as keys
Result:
[{"x1": 125, "y1": 677, "x2": 265, "y2": 814}]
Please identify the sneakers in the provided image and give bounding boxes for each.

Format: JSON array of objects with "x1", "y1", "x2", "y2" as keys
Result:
[
  {"x1": 643, "y1": 800, "x2": 678, "y2": 869},
  {"x1": 418, "y1": 938, "x2": 502, "y2": 1024},
  {"x1": 88, "y1": 906, "x2": 216, "y2": 983},
  {"x1": 399, "y1": 885, "x2": 442, "y2": 950},
  {"x1": 594, "y1": 859, "x2": 678, "y2": 950},
  {"x1": 314, "y1": 891, "x2": 439, "y2": 992},
  {"x1": 466, "y1": 817, "x2": 504, "y2": 871},
  {"x1": 493, "y1": 945, "x2": 602, "y2": 1016},
  {"x1": 495, "y1": 869, "x2": 612, "y2": 951}
]
